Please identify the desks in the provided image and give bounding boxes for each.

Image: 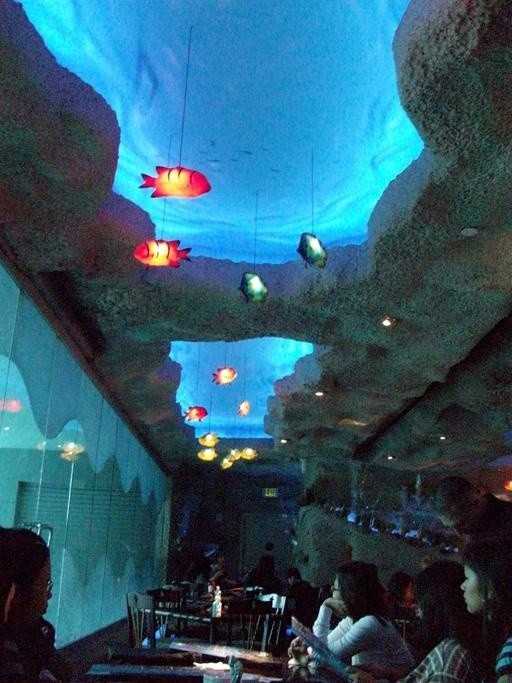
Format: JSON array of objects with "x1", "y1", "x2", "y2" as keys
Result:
[{"x1": 70, "y1": 638, "x2": 291, "y2": 682}]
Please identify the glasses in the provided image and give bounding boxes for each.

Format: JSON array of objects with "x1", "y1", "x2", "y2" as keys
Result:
[
  {"x1": 27, "y1": 577, "x2": 54, "y2": 594},
  {"x1": 330, "y1": 584, "x2": 344, "y2": 591}
]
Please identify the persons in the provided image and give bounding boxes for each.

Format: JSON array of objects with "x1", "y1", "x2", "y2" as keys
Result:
[
  {"x1": 286, "y1": 475, "x2": 512, "y2": 683},
  {"x1": 0, "y1": 525, "x2": 85, "y2": 683},
  {"x1": 174, "y1": 539, "x2": 317, "y2": 628}
]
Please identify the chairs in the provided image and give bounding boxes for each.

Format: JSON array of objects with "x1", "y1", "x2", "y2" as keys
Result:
[{"x1": 126, "y1": 585, "x2": 286, "y2": 654}]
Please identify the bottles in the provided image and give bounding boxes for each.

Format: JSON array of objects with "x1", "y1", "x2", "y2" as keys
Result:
[{"x1": 212, "y1": 586, "x2": 221, "y2": 618}]
